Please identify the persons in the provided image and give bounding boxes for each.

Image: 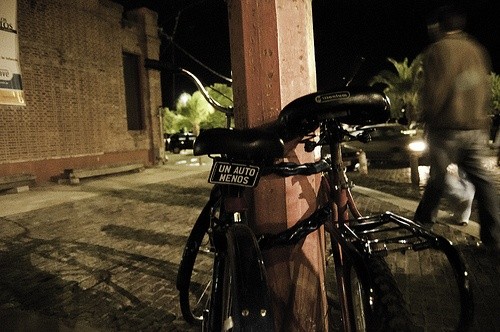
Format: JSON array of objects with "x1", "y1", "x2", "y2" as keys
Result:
[{"x1": 412, "y1": 5, "x2": 500, "y2": 273}]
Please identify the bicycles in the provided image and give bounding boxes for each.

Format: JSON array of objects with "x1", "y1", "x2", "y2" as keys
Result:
[
  {"x1": 143, "y1": 55, "x2": 286, "y2": 332},
  {"x1": 274, "y1": 62, "x2": 440, "y2": 331}
]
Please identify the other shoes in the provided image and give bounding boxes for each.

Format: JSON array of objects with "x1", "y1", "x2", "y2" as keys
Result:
[
  {"x1": 454, "y1": 200, "x2": 469, "y2": 214},
  {"x1": 476, "y1": 240, "x2": 500, "y2": 256},
  {"x1": 449, "y1": 213, "x2": 471, "y2": 227}
]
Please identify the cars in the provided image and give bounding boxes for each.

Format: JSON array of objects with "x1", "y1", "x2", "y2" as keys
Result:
[
  {"x1": 335, "y1": 123, "x2": 430, "y2": 171},
  {"x1": 163, "y1": 127, "x2": 196, "y2": 153}
]
